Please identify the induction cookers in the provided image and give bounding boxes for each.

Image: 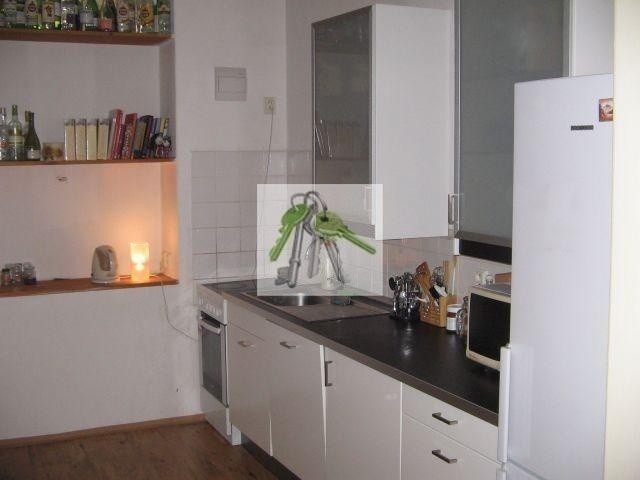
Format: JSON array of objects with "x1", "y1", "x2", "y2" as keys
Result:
[{"x1": 196, "y1": 275, "x2": 310, "y2": 324}]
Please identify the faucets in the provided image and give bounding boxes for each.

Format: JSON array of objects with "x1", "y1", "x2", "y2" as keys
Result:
[{"x1": 304, "y1": 235, "x2": 345, "y2": 293}]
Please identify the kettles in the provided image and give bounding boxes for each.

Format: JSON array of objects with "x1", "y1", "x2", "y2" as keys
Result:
[{"x1": 91, "y1": 245, "x2": 122, "y2": 285}]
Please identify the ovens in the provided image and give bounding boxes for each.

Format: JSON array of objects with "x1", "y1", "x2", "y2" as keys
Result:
[{"x1": 200, "y1": 309, "x2": 232, "y2": 446}]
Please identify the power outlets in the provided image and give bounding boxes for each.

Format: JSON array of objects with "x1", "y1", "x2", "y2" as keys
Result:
[{"x1": 264, "y1": 97, "x2": 276, "y2": 114}]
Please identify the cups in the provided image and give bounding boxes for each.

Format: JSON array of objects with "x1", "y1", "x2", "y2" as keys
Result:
[
  {"x1": 445, "y1": 303, "x2": 464, "y2": 333},
  {"x1": 10, "y1": 264, "x2": 23, "y2": 283}
]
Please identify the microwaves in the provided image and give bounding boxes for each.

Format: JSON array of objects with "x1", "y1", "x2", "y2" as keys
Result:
[{"x1": 465, "y1": 281, "x2": 511, "y2": 374}]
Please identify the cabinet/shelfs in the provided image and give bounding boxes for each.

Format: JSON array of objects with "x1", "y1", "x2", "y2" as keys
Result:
[
  {"x1": 401, "y1": 384, "x2": 498, "y2": 479},
  {"x1": 273, "y1": 323, "x2": 324, "y2": 480},
  {"x1": 448, "y1": 0, "x2": 614, "y2": 253},
  {"x1": 323, "y1": 346, "x2": 401, "y2": 480},
  {"x1": 228, "y1": 304, "x2": 272, "y2": 458},
  {"x1": 0, "y1": 27, "x2": 179, "y2": 445},
  {"x1": 310, "y1": 3, "x2": 455, "y2": 241}
]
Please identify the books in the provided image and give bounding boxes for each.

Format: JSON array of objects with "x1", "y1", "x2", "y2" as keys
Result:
[{"x1": 107, "y1": 108, "x2": 169, "y2": 161}]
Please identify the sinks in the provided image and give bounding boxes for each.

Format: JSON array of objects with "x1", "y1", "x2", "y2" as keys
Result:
[{"x1": 237, "y1": 287, "x2": 391, "y2": 323}]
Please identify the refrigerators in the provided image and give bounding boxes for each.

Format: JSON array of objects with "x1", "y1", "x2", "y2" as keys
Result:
[{"x1": 493, "y1": 70, "x2": 616, "y2": 479}]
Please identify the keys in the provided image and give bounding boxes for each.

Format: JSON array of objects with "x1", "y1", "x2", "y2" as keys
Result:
[{"x1": 268, "y1": 190, "x2": 376, "y2": 290}]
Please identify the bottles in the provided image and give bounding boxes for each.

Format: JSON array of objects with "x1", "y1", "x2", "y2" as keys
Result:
[
  {"x1": 23, "y1": 262, "x2": 37, "y2": 285},
  {"x1": 0, "y1": 0, "x2": 171, "y2": 34},
  {"x1": 2, "y1": 268, "x2": 10, "y2": 286},
  {"x1": 0, "y1": 104, "x2": 43, "y2": 163}
]
